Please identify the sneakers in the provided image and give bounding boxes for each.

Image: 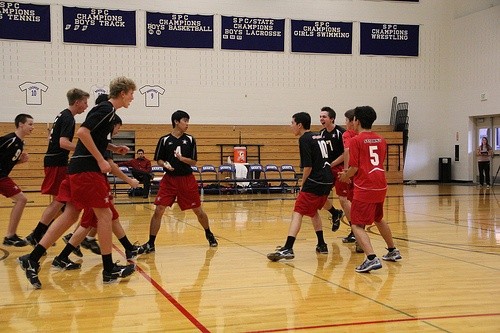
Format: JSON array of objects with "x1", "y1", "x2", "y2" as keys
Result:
[
  {"x1": 382, "y1": 247, "x2": 402, "y2": 262},
  {"x1": 102, "y1": 259, "x2": 135, "y2": 284},
  {"x1": 266, "y1": 246, "x2": 294, "y2": 262},
  {"x1": 19, "y1": 254, "x2": 42, "y2": 289},
  {"x1": 316, "y1": 242, "x2": 328, "y2": 254},
  {"x1": 51, "y1": 256, "x2": 81, "y2": 270},
  {"x1": 26, "y1": 233, "x2": 48, "y2": 256},
  {"x1": 356, "y1": 242, "x2": 364, "y2": 253},
  {"x1": 331, "y1": 209, "x2": 342, "y2": 232},
  {"x1": 81, "y1": 237, "x2": 102, "y2": 255},
  {"x1": 354, "y1": 255, "x2": 382, "y2": 273},
  {"x1": 3, "y1": 233, "x2": 28, "y2": 247},
  {"x1": 342, "y1": 232, "x2": 356, "y2": 242},
  {"x1": 63, "y1": 233, "x2": 83, "y2": 258},
  {"x1": 123, "y1": 240, "x2": 150, "y2": 261}
]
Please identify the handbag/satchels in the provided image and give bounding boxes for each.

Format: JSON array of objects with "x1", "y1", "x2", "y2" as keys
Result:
[{"x1": 127, "y1": 183, "x2": 290, "y2": 195}]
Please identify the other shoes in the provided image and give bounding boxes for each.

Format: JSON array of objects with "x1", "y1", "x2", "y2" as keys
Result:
[
  {"x1": 142, "y1": 247, "x2": 155, "y2": 254},
  {"x1": 486, "y1": 185, "x2": 490, "y2": 190},
  {"x1": 206, "y1": 232, "x2": 218, "y2": 247}
]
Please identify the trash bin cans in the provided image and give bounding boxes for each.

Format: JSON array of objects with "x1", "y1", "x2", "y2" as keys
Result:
[{"x1": 439, "y1": 158, "x2": 451, "y2": 182}]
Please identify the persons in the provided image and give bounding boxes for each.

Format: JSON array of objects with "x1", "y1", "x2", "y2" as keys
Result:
[
  {"x1": 25, "y1": 88, "x2": 150, "y2": 270},
  {"x1": 16, "y1": 77, "x2": 137, "y2": 289},
  {"x1": 320, "y1": 107, "x2": 356, "y2": 243},
  {"x1": 0, "y1": 114, "x2": 34, "y2": 246},
  {"x1": 337, "y1": 106, "x2": 403, "y2": 272},
  {"x1": 476, "y1": 136, "x2": 493, "y2": 189},
  {"x1": 267, "y1": 112, "x2": 333, "y2": 262},
  {"x1": 129, "y1": 149, "x2": 152, "y2": 198},
  {"x1": 137, "y1": 111, "x2": 218, "y2": 254},
  {"x1": 342, "y1": 110, "x2": 365, "y2": 253}
]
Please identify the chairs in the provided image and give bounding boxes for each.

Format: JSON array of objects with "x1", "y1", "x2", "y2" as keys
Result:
[{"x1": 108, "y1": 162, "x2": 304, "y2": 198}]
